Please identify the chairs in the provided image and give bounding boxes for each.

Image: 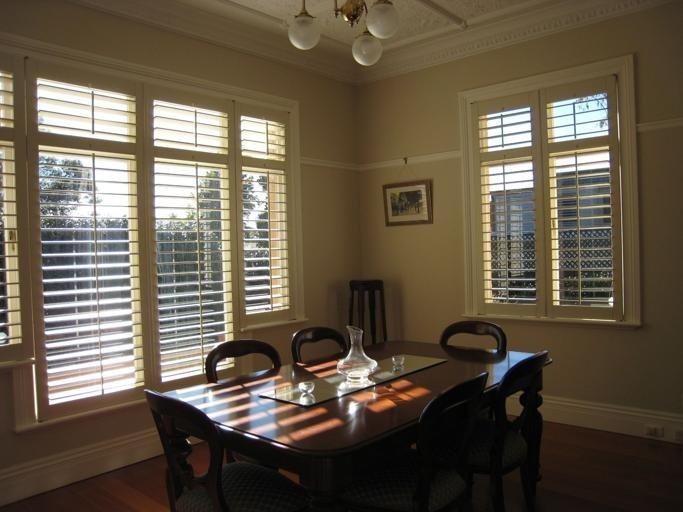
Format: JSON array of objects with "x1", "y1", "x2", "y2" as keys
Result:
[
  {"x1": 289, "y1": 326, "x2": 348, "y2": 363},
  {"x1": 142, "y1": 387, "x2": 311, "y2": 512},
  {"x1": 440, "y1": 349, "x2": 550, "y2": 511},
  {"x1": 204, "y1": 339, "x2": 282, "y2": 384},
  {"x1": 304, "y1": 369, "x2": 490, "y2": 512},
  {"x1": 438, "y1": 319, "x2": 507, "y2": 353}
]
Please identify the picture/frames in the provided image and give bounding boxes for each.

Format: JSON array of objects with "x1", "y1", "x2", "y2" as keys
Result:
[{"x1": 381, "y1": 178, "x2": 434, "y2": 227}]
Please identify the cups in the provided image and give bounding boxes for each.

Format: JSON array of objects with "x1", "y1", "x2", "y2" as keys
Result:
[
  {"x1": 299, "y1": 382, "x2": 314, "y2": 393},
  {"x1": 392, "y1": 356, "x2": 404, "y2": 366},
  {"x1": 300, "y1": 393, "x2": 315, "y2": 402},
  {"x1": 392, "y1": 366, "x2": 404, "y2": 374}
]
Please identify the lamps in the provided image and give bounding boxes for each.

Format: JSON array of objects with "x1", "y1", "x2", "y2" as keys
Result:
[{"x1": 287, "y1": 0, "x2": 400, "y2": 69}]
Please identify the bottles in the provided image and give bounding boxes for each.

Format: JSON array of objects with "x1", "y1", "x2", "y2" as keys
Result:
[{"x1": 337, "y1": 325, "x2": 378, "y2": 379}]
